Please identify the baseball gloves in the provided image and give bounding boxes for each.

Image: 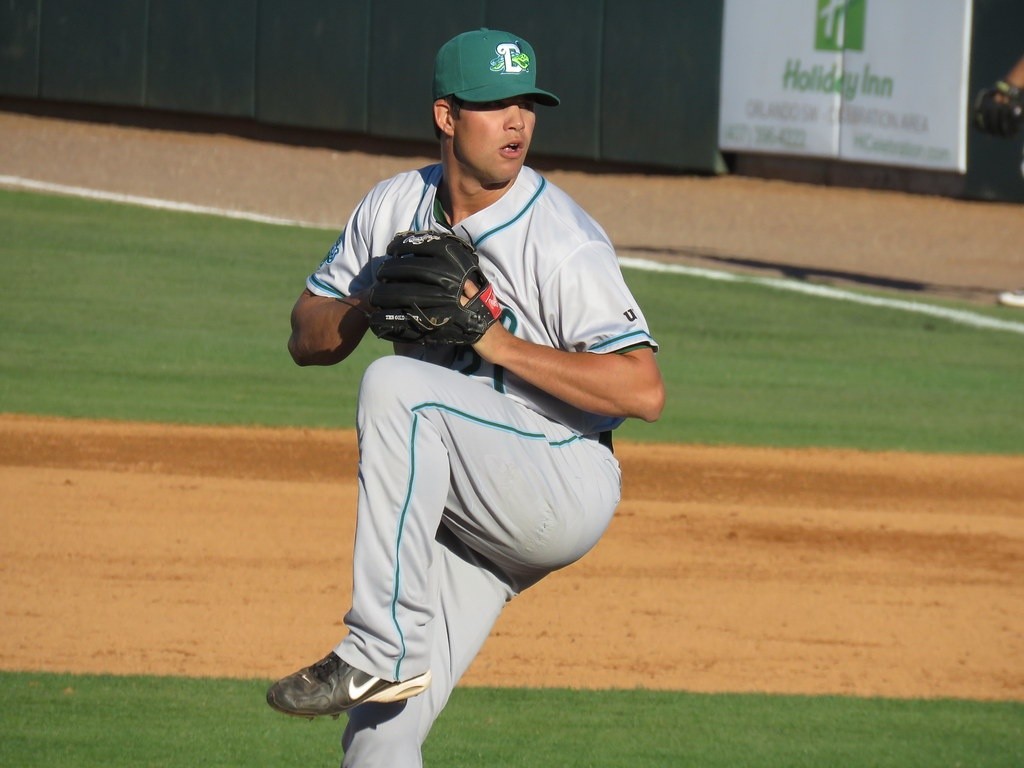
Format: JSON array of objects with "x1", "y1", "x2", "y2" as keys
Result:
[{"x1": 361, "y1": 228, "x2": 500, "y2": 347}]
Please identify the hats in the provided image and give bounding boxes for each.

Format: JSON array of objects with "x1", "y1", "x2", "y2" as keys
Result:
[{"x1": 432, "y1": 27, "x2": 561, "y2": 106}]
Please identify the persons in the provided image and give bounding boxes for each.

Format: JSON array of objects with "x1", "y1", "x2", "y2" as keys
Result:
[
  {"x1": 971, "y1": 57, "x2": 1024, "y2": 138},
  {"x1": 266, "y1": 27, "x2": 666, "y2": 768}
]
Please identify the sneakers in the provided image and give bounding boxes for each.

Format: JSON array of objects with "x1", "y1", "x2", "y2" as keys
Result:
[{"x1": 266, "y1": 651, "x2": 431, "y2": 716}]
